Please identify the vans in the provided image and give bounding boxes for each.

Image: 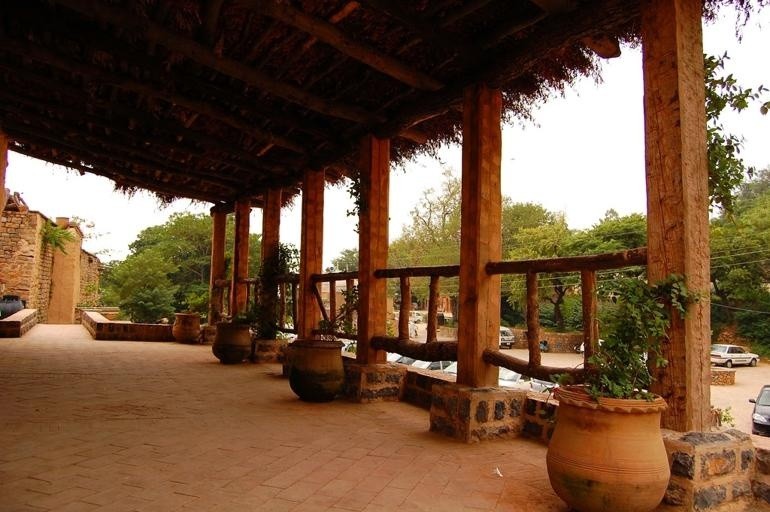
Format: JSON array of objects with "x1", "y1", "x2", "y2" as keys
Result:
[
  {"x1": 499, "y1": 326, "x2": 516, "y2": 350},
  {"x1": 409, "y1": 311, "x2": 423, "y2": 323}
]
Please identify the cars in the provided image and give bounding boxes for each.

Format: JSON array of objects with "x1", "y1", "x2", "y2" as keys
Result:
[
  {"x1": 747, "y1": 384, "x2": 770, "y2": 437},
  {"x1": 709, "y1": 343, "x2": 762, "y2": 367}
]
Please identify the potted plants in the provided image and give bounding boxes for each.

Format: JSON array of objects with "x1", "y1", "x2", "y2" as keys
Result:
[
  {"x1": 288, "y1": 285, "x2": 358, "y2": 402},
  {"x1": 544, "y1": 272, "x2": 704, "y2": 512},
  {"x1": 212, "y1": 242, "x2": 300, "y2": 364},
  {"x1": 172, "y1": 297, "x2": 201, "y2": 344}
]
